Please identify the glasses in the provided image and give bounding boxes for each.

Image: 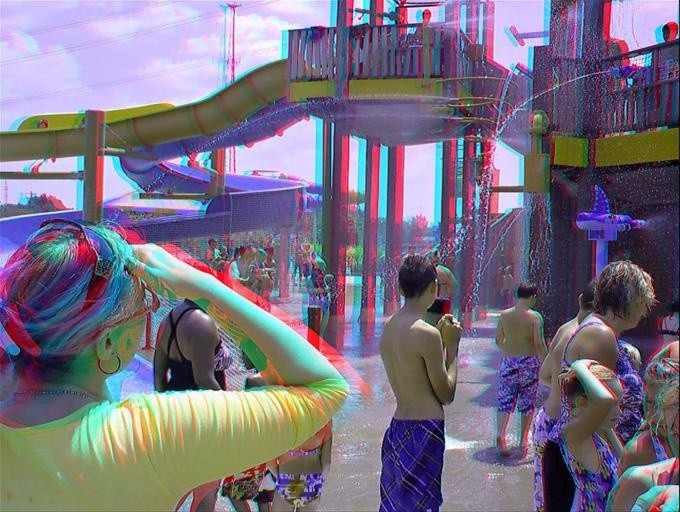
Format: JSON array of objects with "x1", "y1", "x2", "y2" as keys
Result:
[
  {"x1": 82, "y1": 282, "x2": 161, "y2": 343},
  {"x1": 39, "y1": 218, "x2": 114, "y2": 315}
]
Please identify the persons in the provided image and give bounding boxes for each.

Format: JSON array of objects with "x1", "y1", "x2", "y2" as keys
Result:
[
  {"x1": 0, "y1": 219, "x2": 349, "y2": 512},
  {"x1": 415, "y1": 9, "x2": 433, "y2": 40},
  {"x1": 650, "y1": 22, "x2": 679, "y2": 131},
  {"x1": 379, "y1": 247, "x2": 680, "y2": 512}
]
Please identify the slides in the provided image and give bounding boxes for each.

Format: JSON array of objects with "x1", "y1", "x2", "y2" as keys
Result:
[
  {"x1": 279, "y1": 172, "x2": 365, "y2": 210},
  {"x1": 0, "y1": 58, "x2": 311, "y2": 273}
]
